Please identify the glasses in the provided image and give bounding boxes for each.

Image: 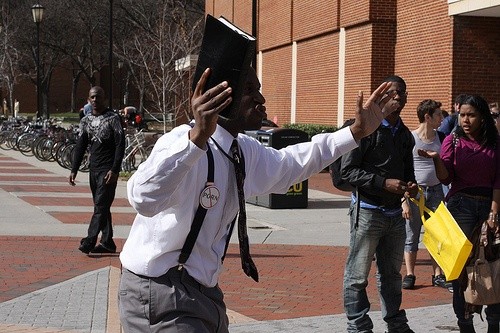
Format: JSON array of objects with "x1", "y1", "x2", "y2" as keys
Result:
[
  {"x1": 386, "y1": 89, "x2": 408, "y2": 98},
  {"x1": 490, "y1": 112, "x2": 500, "y2": 119}
]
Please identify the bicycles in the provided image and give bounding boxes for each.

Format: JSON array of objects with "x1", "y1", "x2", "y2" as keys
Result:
[{"x1": 0, "y1": 115, "x2": 165, "y2": 176}]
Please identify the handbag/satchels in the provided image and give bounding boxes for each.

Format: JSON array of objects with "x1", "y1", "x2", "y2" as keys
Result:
[
  {"x1": 464, "y1": 222, "x2": 500, "y2": 304},
  {"x1": 405, "y1": 185, "x2": 473, "y2": 281}
]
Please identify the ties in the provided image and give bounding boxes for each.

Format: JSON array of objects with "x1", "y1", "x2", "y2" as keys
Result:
[{"x1": 231, "y1": 139, "x2": 259, "y2": 283}]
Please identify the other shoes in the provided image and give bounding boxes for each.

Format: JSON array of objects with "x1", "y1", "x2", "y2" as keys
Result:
[
  {"x1": 489, "y1": 326, "x2": 499, "y2": 333},
  {"x1": 402, "y1": 274, "x2": 416, "y2": 289},
  {"x1": 79, "y1": 238, "x2": 93, "y2": 254},
  {"x1": 431, "y1": 275, "x2": 452, "y2": 288},
  {"x1": 463, "y1": 324, "x2": 475, "y2": 333},
  {"x1": 389, "y1": 328, "x2": 415, "y2": 333},
  {"x1": 90, "y1": 245, "x2": 116, "y2": 254}
]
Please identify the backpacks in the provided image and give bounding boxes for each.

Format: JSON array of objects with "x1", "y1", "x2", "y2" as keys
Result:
[{"x1": 329, "y1": 118, "x2": 407, "y2": 191}]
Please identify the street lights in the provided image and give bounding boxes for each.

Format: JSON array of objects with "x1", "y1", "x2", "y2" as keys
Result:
[{"x1": 30, "y1": 4, "x2": 44, "y2": 125}]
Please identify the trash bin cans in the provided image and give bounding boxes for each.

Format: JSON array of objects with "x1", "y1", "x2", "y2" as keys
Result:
[{"x1": 244, "y1": 127, "x2": 309, "y2": 210}]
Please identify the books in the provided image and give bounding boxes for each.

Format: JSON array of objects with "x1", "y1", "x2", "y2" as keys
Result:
[{"x1": 192, "y1": 14, "x2": 257, "y2": 119}]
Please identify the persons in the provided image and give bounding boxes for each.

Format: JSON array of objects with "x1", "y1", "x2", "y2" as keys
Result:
[
  {"x1": 120, "y1": 103, "x2": 141, "y2": 126},
  {"x1": 68, "y1": 86, "x2": 124, "y2": 253},
  {"x1": 340, "y1": 76, "x2": 419, "y2": 333},
  {"x1": 2, "y1": 97, "x2": 19, "y2": 119},
  {"x1": 83, "y1": 98, "x2": 92, "y2": 115},
  {"x1": 418, "y1": 95, "x2": 500, "y2": 333},
  {"x1": 436, "y1": 94, "x2": 500, "y2": 194},
  {"x1": 118, "y1": 63, "x2": 400, "y2": 333},
  {"x1": 403, "y1": 99, "x2": 445, "y2": 288}
]
